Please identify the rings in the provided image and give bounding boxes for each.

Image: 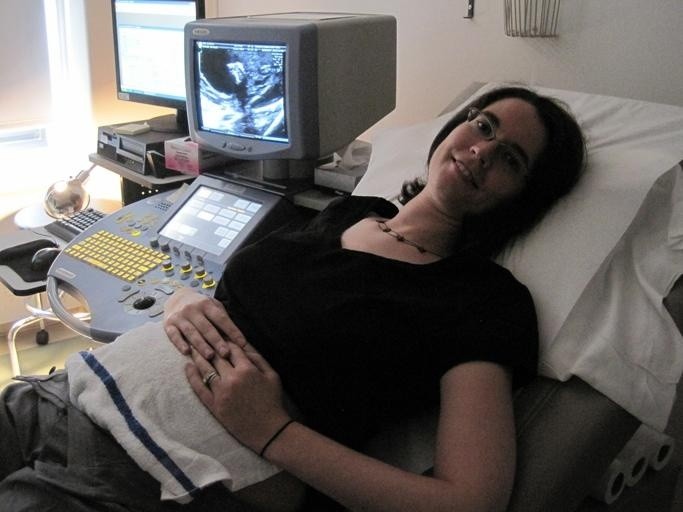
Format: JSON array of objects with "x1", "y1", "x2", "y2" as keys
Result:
[{"x1": 201, "y1": 371, "x2": 218, "y2": 387}]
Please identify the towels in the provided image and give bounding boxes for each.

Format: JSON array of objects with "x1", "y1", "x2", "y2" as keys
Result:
[{"x1": 66, "y1": 314, "x2": 288, "y2": 503}]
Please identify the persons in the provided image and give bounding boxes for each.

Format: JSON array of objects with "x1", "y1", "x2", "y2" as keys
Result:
[{"x1": 0, "y1": 86, "x2": 585, "y2": 510}]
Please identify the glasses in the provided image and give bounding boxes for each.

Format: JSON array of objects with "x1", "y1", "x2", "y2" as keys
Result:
[{"x1": 467, "y1": 104, "x2": 528, "y2": 179}]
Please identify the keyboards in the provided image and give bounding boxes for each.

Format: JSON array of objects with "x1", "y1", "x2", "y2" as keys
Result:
[{"x1": 43, "y1": 208, "x2": 107, "y2": 243}]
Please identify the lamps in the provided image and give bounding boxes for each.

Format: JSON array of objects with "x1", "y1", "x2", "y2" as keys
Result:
[{"x1": 45, "y1": 156, "x2": 100, "y2": 222}]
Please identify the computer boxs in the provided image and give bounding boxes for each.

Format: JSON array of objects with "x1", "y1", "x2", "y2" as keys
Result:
[{"x1": 97, "y1": 114, "x2": 190, "y2": 176}]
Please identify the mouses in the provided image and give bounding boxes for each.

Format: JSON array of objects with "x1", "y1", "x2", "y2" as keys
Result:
[{"x1": 31, "y1": 248, "x2": 62, "y2": 272}]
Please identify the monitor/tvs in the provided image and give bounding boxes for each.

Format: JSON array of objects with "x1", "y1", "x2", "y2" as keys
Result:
[
  {"x1": 184, "y1": 14, "x2": 397, "y2": 195},
  {"x1": 111, "y1": 0, "x2": 205, "y2": 134}
]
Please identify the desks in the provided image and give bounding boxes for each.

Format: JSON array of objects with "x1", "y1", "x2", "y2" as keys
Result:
[
  {"x1": 0, "y1": 204, "x2": 81, "y2": 380},
  {"x1": 86, "y1": 136, "x2": 370, "y2": 226}
]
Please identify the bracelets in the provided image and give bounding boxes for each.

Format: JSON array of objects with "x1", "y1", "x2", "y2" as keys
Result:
[{"x1": 259, "y1": 418, "x2": 295, "y2": 456}]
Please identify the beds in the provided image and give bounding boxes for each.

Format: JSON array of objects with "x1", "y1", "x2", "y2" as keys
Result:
[{"x1": 177, "y1": 76, "x2": 682, "y2": 510}]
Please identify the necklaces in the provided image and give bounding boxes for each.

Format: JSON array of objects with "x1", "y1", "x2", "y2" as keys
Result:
[{"x1": 375, "y1": 218, "x2": 446, "y2": 260}]
